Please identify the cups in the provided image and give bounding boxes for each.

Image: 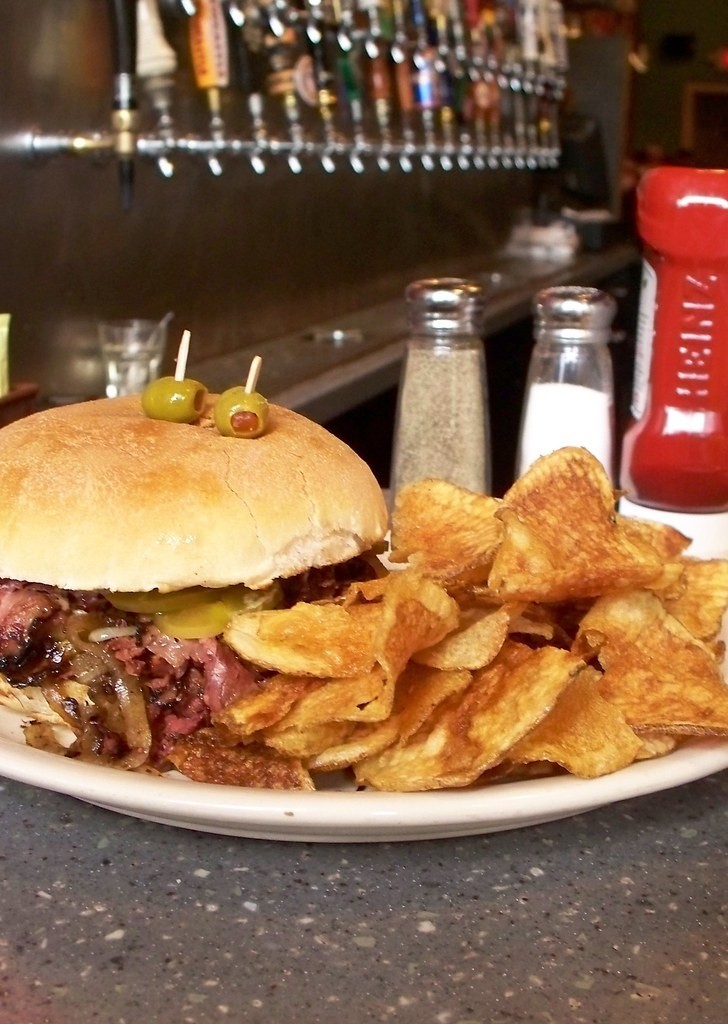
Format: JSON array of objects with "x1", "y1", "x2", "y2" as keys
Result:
[{"x1": 100, "y1": 319, "x2": 167, "y2": 398}]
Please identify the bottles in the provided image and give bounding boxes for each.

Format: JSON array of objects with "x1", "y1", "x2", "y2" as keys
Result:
[
  {"x1": 517, "y1": 287, "x2": 616, "y2": 489},
  {"x1": 391, "y1": 276, "x2": 490, "y2": 521}
]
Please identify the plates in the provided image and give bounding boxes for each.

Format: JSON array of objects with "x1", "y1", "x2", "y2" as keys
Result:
[{"x1": 0, "y1": 703, "x2": 728, "y2": 842}]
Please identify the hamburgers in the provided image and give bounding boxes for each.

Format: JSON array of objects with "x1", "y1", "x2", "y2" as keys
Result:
[{"x1": 0, "y1": 381, "x2": 389, "y2": 774}]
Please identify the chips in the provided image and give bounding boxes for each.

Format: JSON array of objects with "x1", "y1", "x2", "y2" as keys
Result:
[{"x1": 172, "y1": 446, "x2": 728, "y2": 790}]
